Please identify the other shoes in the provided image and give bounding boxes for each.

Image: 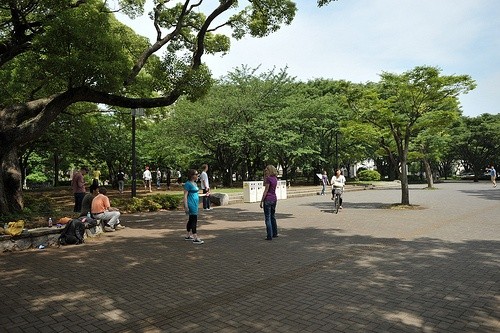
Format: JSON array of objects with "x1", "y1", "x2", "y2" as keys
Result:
[
  {"x1": 208, "y1": 208, "x2": 213, "y2": 209},
  {"x1": 265, "y1": 238, "x2": 272, "y2": 240},
  {"x1": 339, "y1": 205, "x2": 342, "y2": 209},
  {"x1": 203, "y1": 208, "x2": 210, "y2": 210},
  {"x1": 332, "y1": 197, "x2": 335, "y2": 200}
]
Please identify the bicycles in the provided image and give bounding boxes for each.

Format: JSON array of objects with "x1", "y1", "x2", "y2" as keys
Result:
[{"x1": 331, "y1": 184, "x2": 343, "y2": 214}]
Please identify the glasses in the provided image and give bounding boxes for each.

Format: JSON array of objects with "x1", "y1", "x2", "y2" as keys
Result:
[{"x1": 193, "y1": 173, "x2": 199, "y2": 176}]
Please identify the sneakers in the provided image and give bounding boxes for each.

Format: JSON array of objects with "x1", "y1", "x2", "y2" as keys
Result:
[
  {"x1": 185, "y1": 236, "x2": 194, "y2": 240},
  {"x1": 115, "y1": 224, "x2": 125, "y2": 230},
  {"x1": 192, "y1": 238, "x2": 204, "y2": 244},
  {"x1": 104, "y1": 226, "x2": 115, "y2": 232}
]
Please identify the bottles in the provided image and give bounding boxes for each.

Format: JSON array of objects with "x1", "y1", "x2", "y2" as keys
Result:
[
  {"x1": 4, "y1": 222, "x2": 7, "y2": 228},
  {"x1": 49, "y1": 217, "x2": 52, "y2": 227},
  {"x1": 87, "y1": 212, "x2": 90, "y2": 218}
]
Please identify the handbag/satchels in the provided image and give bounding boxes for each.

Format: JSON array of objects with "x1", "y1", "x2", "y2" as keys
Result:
[
  {"x1": 4, "y1": 219, "x2": 24, "y2": 236},
  {"x1": 79, "y1": 216, "x2": 98, "y2": 228}
]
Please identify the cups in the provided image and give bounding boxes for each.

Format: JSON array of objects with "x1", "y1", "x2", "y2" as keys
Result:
[{"x1": 57, "y1": 223, "x2": 62, "y2": 228}]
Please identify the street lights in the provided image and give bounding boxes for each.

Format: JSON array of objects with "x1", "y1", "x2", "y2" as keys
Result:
[
  {"x1": 131, "y1": 107, "x2": 144, "y2": 196},
  {"x1": 335, "y1": 132, "x2": 342, "y2": 175}
]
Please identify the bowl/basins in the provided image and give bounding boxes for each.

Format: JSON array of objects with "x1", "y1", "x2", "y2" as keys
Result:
[{"x1": 22, "y1": 229, "x2": 28, "y2": 233}]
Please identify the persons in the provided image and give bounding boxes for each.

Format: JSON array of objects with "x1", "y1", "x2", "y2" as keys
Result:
[
  {"x1": 201, "y1": 164, "x2": 213, "y2": 210},
  {"x1": 183, "y1": 169, "x2": 204, "y2": 244},
  {"x1": 117, "y1": 171, "x2": 125, "y2": 193},
  {"x1": 176, "y1": 168, "x2": 182, "y2": 186},
  {"x1": 143, "y1": 166, "x2": 153, "y2": 193},
  {"x1": 143, "y1": 165, "x2": 150, "y2": 171},
  {"x1": 488, "y1": 166, "x2": 496, "y2": 188},
  {"x1": 157, "y1": 167, "x2": 161, "y2": 189},
  {"x1": 321, "y1": 171, "x2": 330, "y2": 196},
  {"x1": 73, "y1": 166, "x2": 89, "y2": 218},
  {"x1": 92, "y1": 167, "x2": 101, "y2": 186},
  {"x1": 260, "y1": 165, "x2": 278, "y2": 241},
  {"x1": 331, "y1": 168, "x2": 346, "y2": 209},
  {"x1": 81, "y1": 184, "x2": 125, "y2": 231},
  {"x1": 436, "y1": 174, "x2": 439, "y2": 179},
  {"x1": 74, "y1": 166, "x2": 79, "y2": 176}
]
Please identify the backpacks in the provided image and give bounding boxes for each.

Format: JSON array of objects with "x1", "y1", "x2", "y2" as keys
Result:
[{"x1": 58, "y1": 219, "x2": 85, "y2": 245}]
